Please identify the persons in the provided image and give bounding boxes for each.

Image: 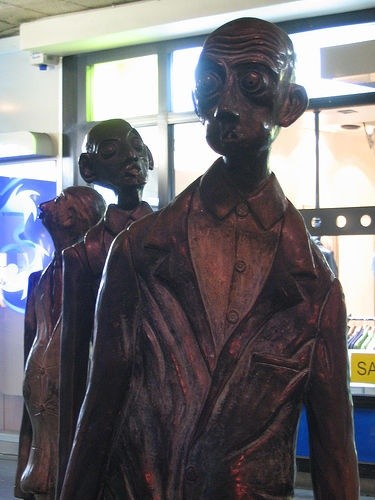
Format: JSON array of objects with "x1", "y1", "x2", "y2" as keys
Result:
[
  {"x1": 54, "y1": 118, "x2": 156, "y2": 500},
  {"x1": 13, "y1": 184, "x2": 107, "y2": 499},
  {"x1": 55, "y1": 16, "x2": 362, "y2": 500}
]
